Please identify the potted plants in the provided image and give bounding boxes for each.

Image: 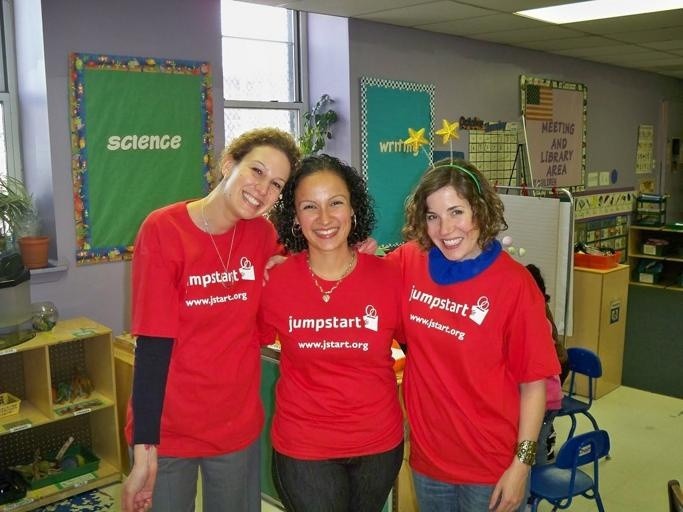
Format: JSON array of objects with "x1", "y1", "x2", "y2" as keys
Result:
[{"x1": 17, "y1": 194, "x2": 49, "y2": 270}]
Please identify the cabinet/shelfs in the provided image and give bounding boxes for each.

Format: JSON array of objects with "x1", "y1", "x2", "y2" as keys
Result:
[
  {"x1": 0, "y1": 316, "x2": 123, "y2": 512},
  {"x1": 626, "y1": 223, "x2": 683, "y2": 292},
  {"x1": 633, "y1": 193, "x2": 668, "y2": 227},
  {"x1": 561, "y1": 264, "x2": 630, "y2": 400}
]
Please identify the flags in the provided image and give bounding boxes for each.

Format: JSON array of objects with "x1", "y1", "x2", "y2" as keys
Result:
[{"x1": 525, "y1": 84, "x2": 555, "y2": 124}]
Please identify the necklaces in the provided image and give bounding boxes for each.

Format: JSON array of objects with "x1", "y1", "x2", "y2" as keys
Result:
[
  {"x1": 303, "y1": 247, "x2": 354, "y2": 303},
  {"x1": 199, "y1": 196, "x2": 236, "y2": 290}
]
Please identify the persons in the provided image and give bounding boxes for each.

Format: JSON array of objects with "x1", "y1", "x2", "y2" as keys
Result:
[
  {"x1": 526, "y1": 317, "x2": 563, "y2": 506},
  {"x1": 254, "y1": 153, "x2": 410, "y2": 512},
  {"x1": 259, "y1": 155, "x2": 565, "y2": 512},
  {"x1": 524, "y1": 263, "x2": 569, "y2": 366},
  {"x1": 117, "y1": 126, "x2": 378, "y2": 512}
]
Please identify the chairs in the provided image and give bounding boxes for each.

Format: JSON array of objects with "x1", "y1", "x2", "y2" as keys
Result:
[
  {"x1": 553, "y1": 347, "x2": 611, "y2": 460},
  {"x1": 668, "y1": 479, "x2": 683, "y2": 512},
  {"x1": 527, "y1": 430, "x2": 610, "y2": 511}
]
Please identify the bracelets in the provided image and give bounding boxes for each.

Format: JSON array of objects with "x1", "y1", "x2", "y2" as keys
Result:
[{"x1": 515, "y1": 438, "x2": 537, "y2": 469}]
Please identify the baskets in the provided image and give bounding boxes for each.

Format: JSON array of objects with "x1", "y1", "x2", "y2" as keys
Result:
[{"x1": 0, "y1": 393, "x2": 21, "y2": 419}]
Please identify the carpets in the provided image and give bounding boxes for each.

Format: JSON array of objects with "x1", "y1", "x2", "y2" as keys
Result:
[{"x1": 621, "y1": 283, "x2": 683, "y2": 400}]
